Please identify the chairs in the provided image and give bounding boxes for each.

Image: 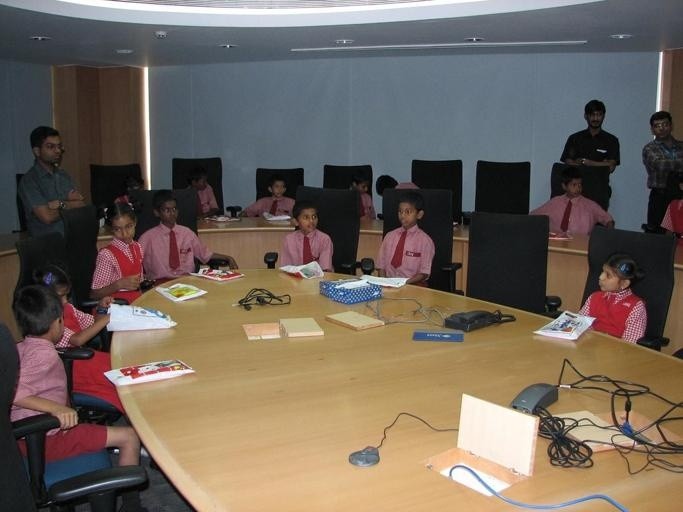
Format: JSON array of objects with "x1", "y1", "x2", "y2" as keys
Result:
[
  {"x1": 323, "y1": 164, "x2": 372, "y2": 198},
  {"x1": 0, "y1": 373, "x2": 154, "y2": 512},
  {"x1": 234, "y1": 168, "x2": 304, "y2": 218},
  {"x1": 556, "y1": 225, "x2": 675, "y2": 351},
  {"x1": 171, "y1": 158, "x2": 234, "y2": 219},
  {"x1": 466, "y1": 213, "x2": 561, "y2": 317},
  {"x1": 361, "y1": 188, "x2": 463, "y2": 295},
  {"x1": 12, "y1": 232, "x2": 125, "y2": 427},
  {"x1": 90, "y1": 164, "x2": 142, "y2": 208},
  {"x1": 377, "y1": 160, "x2": 463, "y2": 225},
  {"x1": 131, "y1": 188, "x2": 228, "y2": 270},
  {"x1": 60, "y1": 206, "x2": 156, "y2": 316},
  {"x1": 0, "y1": 322, "x2": 150, "y2": 505},
  {"x1": 464, "y1": 160, "x2": 530, "y2": 225},
  {"x1": 12, "y1": 173, "x2": 28, "y2": 233},
  {"x1": 665, "y1": 171, "x2": 683, "y2": 215},
  {"x1": 551, "y1": 163, "x2": 609, "y2": 213},
  {"x1": 264, "y1": 185, "x2": 362, "y2": 276}
]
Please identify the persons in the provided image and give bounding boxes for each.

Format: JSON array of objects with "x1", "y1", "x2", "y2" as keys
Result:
[
  {"x1": 561, "y1": 99, "x2": 620, "y2": 197},
  {"x1": 89, "y1": 196, "x2": 145, "y2": 305},
  {"x1": 528, "y1": 167, "x2": 615, "y2": 235},
  {"x1": 376, "y1": 175, "x2": 419, "y2": 197},
  {"x1": 661, "y1": 173, "x2": 683, "y2": 238},
  {"x1": 239, "y1": 176, "x2": 295, "y2": 217},
  {"x1": 138, "y1": 191, "x2": 238, "y2": 288},
  {"x1": 115, "y1": 176, "x2": 144, "y2": 204},
  {"x1": 642, "y1": 110, "x2": 683, "y2": 226},
  {"x1": 347, "y1": 174, "x2": 377, "y2": 221},
  {"x1": 18, "y1": 125, "x2": 87, "y2": 237},
  {"x1": 577, "y1": 251, "x2": 648, "y2": 345},
  {"x1": 375, "y1": 191, "x2": 435, "y2": 287},
  {"x1": 186, "y1": 164, "x2": 220, "y2": 220},
  {"x1": 30, "y1": 266, "x2": 125, "y2": 415},
  {"x1": 7, "y1": 286, "x2": 149, "y2": 512},
  {"x1": 280, "y1": 199, "x2": 333, "y2": 272}
]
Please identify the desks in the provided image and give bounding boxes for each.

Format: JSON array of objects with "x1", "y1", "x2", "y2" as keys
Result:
[{"x1": 0, "y1": 218, "x2": 683, "y2": 345}]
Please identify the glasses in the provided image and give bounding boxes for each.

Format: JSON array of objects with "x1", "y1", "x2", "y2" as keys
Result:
[
  {"x1": 653, "y1": 121, "x2": 670, "y2": 128},
  {"x1": 45, "y1": 141, "x2": 66, "y2": 150}
]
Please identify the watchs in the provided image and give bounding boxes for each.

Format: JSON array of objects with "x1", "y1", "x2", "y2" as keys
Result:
[
  {"x1": 580, "y1": 158, "x2": 586, "y2": 165},
  {"x1": 59, "y1": 200, "x2": 66, "y2": 210}
]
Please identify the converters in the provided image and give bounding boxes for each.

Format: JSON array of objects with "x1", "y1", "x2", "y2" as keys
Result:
[{"x1": 510, "y1": 383, "x2": 559, "y2": 415}]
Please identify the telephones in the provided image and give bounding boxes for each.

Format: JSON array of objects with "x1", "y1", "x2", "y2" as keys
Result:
[{"x1": 444, "y1": 309, "x2": 518, "y2": 333}]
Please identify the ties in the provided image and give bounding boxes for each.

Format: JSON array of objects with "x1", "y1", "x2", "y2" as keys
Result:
[
  {"x1": 303, "y1": 236, "x2": 313, "y2": 263},
  {"x1": 270, "y1": 199, "x2": 278, "y2": 215},
  {"x1": 391, "y1": 232, "x2": 407, "y2": 269},
  {"x1": 167, "y1": 230, "x2": 181, "y2": 272},
  {"x1": 561, "y1": 200, "x2": 573, "y2": 233}
]
